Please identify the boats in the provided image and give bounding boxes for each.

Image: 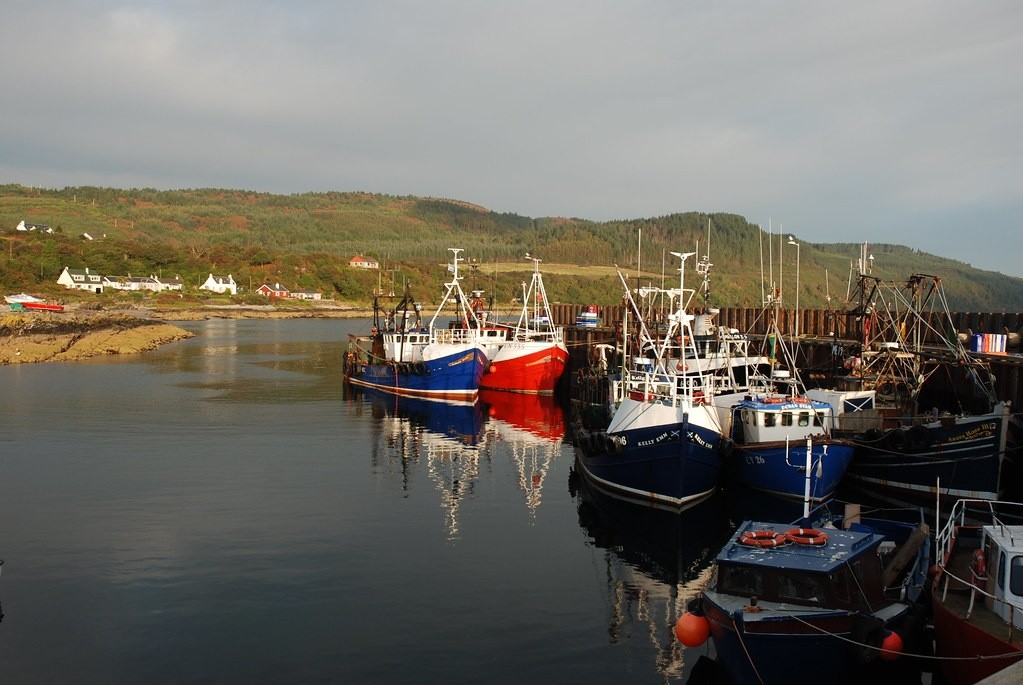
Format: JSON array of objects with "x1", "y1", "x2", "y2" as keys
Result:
[
  {"x1": 337, "y1": 216, "x2": 1023, "y2": 685},
  {"x1": 3, "y1": 292, "x2": 47, "y2": 304},
  {"x1": 20, "y1": 301, "x2": 64, "y2": 313}
]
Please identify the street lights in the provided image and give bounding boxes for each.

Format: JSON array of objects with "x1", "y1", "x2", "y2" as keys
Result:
[{"x1": 788, "y1": 241, "x2": 800, "y2": 337}]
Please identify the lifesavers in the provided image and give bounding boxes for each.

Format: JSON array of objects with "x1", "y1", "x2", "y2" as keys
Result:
[
  {"x1": 604, "y1": 436, "x2": 622, "y2": 457},
  {"x1": 595, "y1": 432, "x2": 606, "y2": 452},
  {"x1": 785, "y1": 529, "x2": 828, "y2": 545},
  {"x1": 787, "y1": 397, "x2": 809, "y2": 402},
  {"x1": 582, "y1": 435, "x2": 591, "y2": 455},
  {"x1": 589, "y1": 433, "x2": 599, "y2": 454},
  {"x1": 414, "y1": 361, "x2": 426, "y2": 375},
  {"x1": 762, "y1": 398, "x2": 784, "y2": 403},
  {"x1": 570, "y1": 428, "x2": 589, "y2": 449},
  {"x1": 390, "y1": 362, "x2": 413, "y2": 375},
  {"x1": 740, "y1": 530, "x2": 786, "y2": 546},
  {"x1": 971, "y1": 549, "x2": 986, "y2": 598}
]
